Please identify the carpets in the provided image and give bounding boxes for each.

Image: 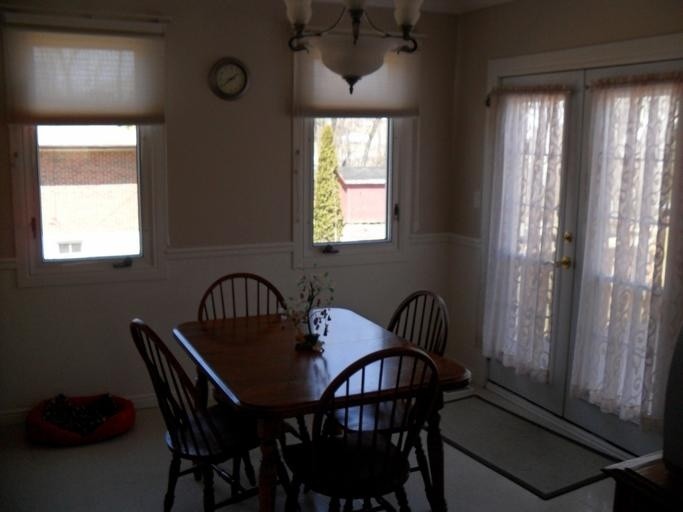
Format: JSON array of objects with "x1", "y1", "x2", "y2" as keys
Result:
[{"x1": 423, "y1": 394, "x2": 623, "y2": 501}]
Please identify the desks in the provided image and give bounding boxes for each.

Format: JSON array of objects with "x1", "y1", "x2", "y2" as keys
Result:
[{"x1": 170, "y1": 306, "x2": 472, "y2": 512}]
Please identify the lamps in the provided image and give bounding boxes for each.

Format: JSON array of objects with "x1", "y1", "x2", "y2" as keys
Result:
[{"x1": 284, "y1": 0, "x2": 423, "y2": 95}]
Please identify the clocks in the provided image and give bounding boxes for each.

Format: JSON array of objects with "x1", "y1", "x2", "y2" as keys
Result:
[{"x1": 209, "y1": 56, "x2": 251, "y2": 101}]
